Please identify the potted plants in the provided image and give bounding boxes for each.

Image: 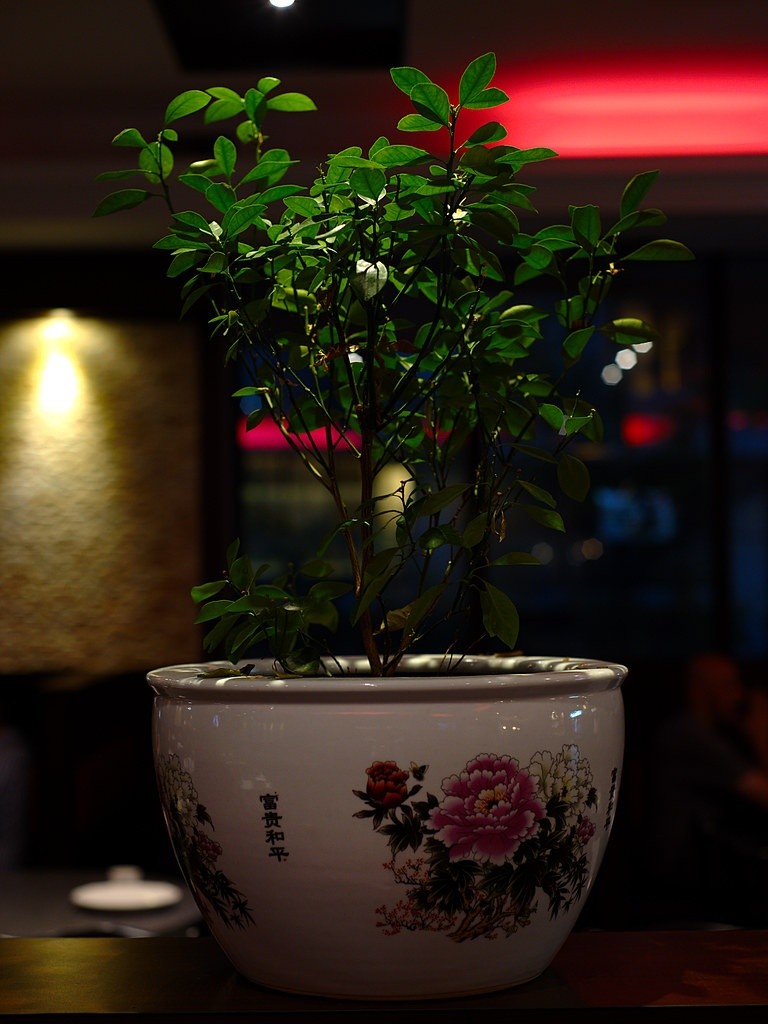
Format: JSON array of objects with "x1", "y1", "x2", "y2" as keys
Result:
[{"x1": 114, "y1": 54, "x2": 675, "y2": 1011}]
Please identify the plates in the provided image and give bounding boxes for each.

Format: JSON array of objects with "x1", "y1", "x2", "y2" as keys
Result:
[{"x1": 67, "y1": 880, "x2": 183, "y2": 912}]
